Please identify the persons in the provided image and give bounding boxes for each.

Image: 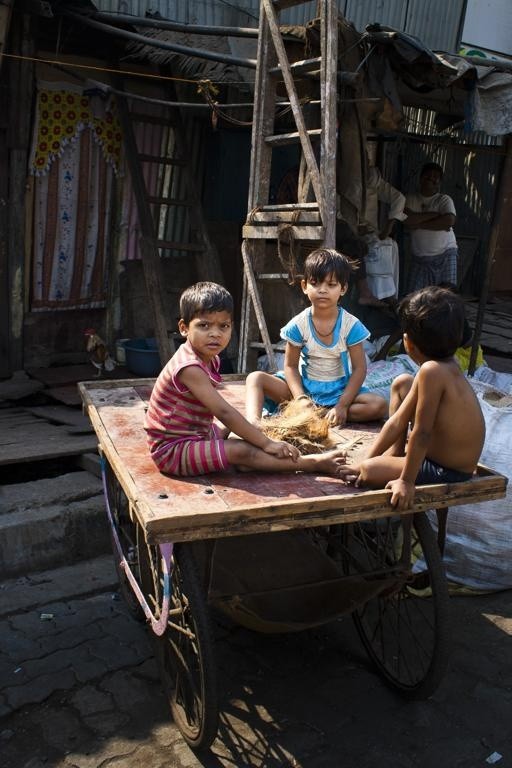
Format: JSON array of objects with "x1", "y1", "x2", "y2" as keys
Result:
[
  {"x1": 141, "y1": 279, "x2": 348, "y2": 478},
  {"x1": 374, "y1": 280, "x2": 484, "y2": 373},
  {"x1": 341, "y1": 146, "x2": 407, "y2": 301},
  {"x1": 401, "y1": 162, "x2": 459, "y2": 296},
  {"x1": 243, "y1": 247, "x2": 388, "y2": 428},
  {"x1": 335, "y1": 284, "x2": 488, "y2": 512}
]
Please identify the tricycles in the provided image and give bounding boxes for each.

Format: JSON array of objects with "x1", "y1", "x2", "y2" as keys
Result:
[{"x1": 72, "y1": 372, "x2": 509, "y2": 754}]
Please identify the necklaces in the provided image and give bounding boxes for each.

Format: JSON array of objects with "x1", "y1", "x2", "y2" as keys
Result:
[{"x1": 312, "y1": 320, "x2": 336, "y2": 337}]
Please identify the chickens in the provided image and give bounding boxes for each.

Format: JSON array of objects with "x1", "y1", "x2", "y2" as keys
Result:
[{"x1": 82, "y1": 326, "x2": 131, "y2": 379}]
